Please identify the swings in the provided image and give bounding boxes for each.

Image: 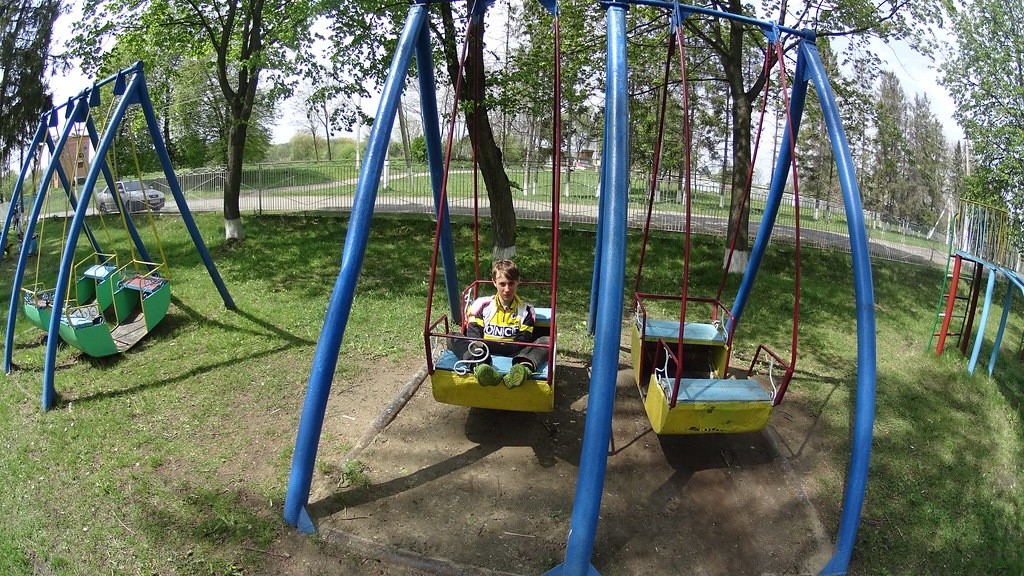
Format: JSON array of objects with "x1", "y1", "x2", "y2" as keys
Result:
[
  {"x1": 423, "y1": 1, "x2": 562, "y2": 414},
  {"x1": 632, "y1": 3, "x2": 800, "y2": 436},
  {"x1": 20, "y1": 93, "x2": 172, "y2": 357}
]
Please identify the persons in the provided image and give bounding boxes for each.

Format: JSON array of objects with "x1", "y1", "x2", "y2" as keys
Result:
[
  {"x1": 445, "y1": 260, "x2": 550, "y2": 389},
  {"x1": 13, "y1": 204, "x2": 19, "y2": 230}
]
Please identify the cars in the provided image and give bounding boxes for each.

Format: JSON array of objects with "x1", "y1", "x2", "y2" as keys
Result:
[{"x1": 94, "y1": 180, "x2": 165, "y2": 213}]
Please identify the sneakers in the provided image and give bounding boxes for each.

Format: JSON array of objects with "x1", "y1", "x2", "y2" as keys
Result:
[
  {"x1": 502, "y1": 363, "x2": 531, "y2": 390},
  {"x1": 473, "y1": 364, "x2": 503, "y2": 387}
]
